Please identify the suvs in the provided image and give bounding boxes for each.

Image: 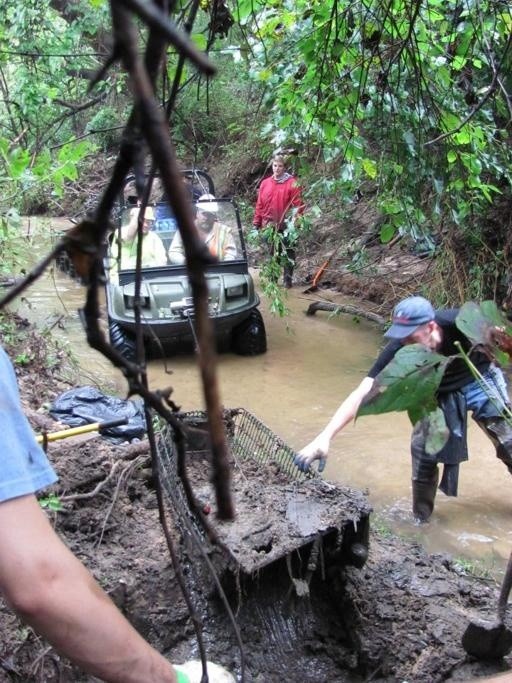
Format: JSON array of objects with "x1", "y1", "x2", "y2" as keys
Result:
[{"x1": 96, "y1": 167, "x2": 267, "y2": 364}]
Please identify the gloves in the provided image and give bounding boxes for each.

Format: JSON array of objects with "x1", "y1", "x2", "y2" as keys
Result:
[{"x1": 170, "y1": 661, "x2": 236, "y2": 683}]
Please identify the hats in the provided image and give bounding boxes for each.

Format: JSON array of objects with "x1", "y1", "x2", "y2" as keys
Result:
[{"x1": 382, "y1": 296, "x2": 435, "y2": 342}]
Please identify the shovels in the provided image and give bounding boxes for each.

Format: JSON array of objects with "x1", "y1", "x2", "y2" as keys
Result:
[{"x1": 302, "y1": 243, "x2": 343, "y2": 293}]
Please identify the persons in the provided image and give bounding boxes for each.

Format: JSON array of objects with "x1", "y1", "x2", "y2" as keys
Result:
[
  {"x1": 251, "y1": 154, "x2": 307, "y2": 290},
  {"x1": 1, "y1": 343, "x2": 240, "y2": 683},
  {"x1": 110, "y1": 203, "x2": 168, "y2": 271},
  {"x1": 292, "y1": 294, "x2": 512, "y2": 526},
  {"x1": 167, "y1": 193, "x2": 238, "y2": 266}
]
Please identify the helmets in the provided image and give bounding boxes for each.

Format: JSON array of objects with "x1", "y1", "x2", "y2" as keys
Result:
[
  {"x1": 130, "y1": 205, "x2": 156, "y2": 221},
  {"x1": 196, "y1": 193, "x2": 220, "y2": 213}
]
